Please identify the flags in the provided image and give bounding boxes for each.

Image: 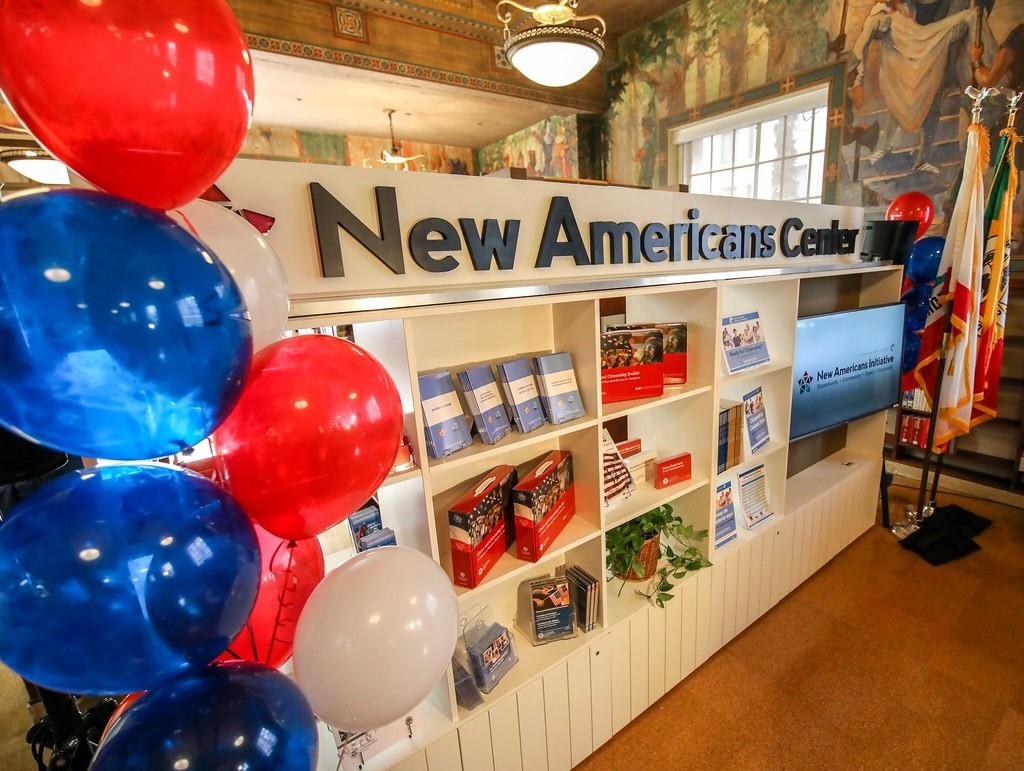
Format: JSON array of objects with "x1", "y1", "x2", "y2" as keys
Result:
[{"x1": 915, "y1": 121, "x2": 1022, "y2": 457}]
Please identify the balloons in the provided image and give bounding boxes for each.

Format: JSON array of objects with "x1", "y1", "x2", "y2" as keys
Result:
[
  {"x1": 1, "y1": 0, "x2": 460, "y2": 771},
  {"x1": 878, "y1": 193, "x2": 945, "y2": 393}
]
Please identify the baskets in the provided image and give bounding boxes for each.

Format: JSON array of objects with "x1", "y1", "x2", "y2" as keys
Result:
[{"x1": 610, "y1": 528, "x2": 660, "y2": 582}]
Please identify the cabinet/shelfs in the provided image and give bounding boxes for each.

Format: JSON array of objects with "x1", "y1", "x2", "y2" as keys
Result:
[{"x1": 286, "y1": 261, "x2": 908, "y2": 771}]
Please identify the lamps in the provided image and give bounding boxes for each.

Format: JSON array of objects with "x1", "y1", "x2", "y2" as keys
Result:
[{"x1": 496, "y1": 0, "x2": 607, "y2": 88}]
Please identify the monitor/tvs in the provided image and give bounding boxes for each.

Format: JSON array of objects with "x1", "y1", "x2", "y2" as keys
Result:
[{"x1": 789, "y1": 299, "x2": 907, "y2": 445}]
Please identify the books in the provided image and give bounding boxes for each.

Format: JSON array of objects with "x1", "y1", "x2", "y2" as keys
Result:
[{"x1": 289, "y1": 309, "x2": 776, "y2": 760}]
